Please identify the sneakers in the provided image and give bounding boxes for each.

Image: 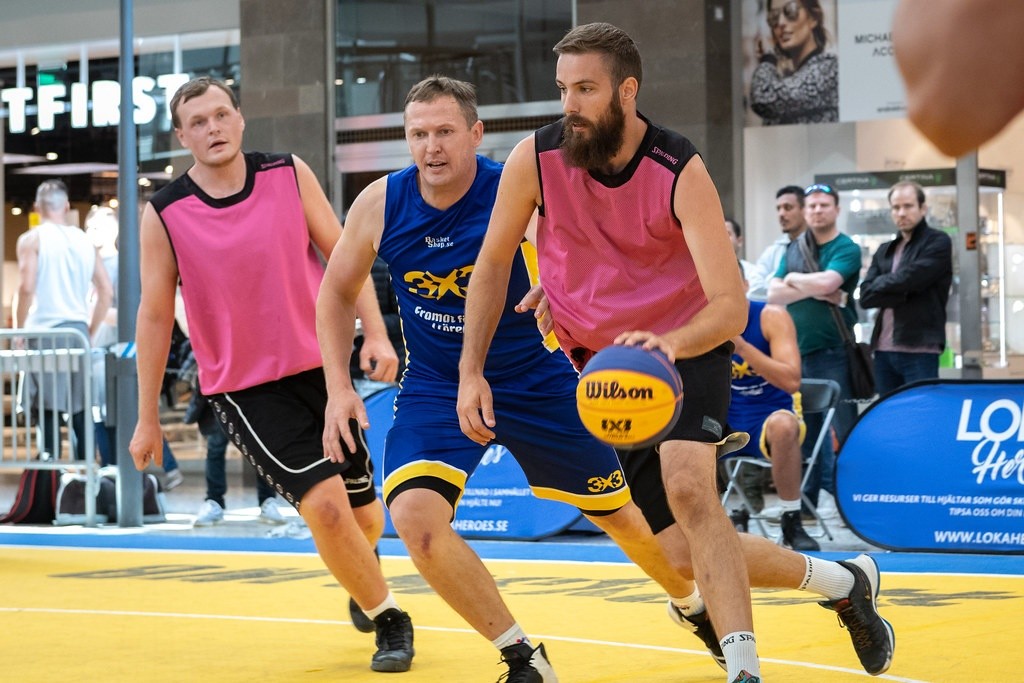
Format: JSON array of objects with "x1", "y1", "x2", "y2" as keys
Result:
[
  {"x1": 817, "y1": 553, "x2": 895, "y2": 676},
  {"x1": 259, "y1": 498, "x2": 286, "y2": 526},
  {"x1": 370, "y1": 607, "x2": 415, "y2": 672},
  {"x1": 348, "y1": 546, "x2": 381, "y2": 633},
  {"x1": 496, "y1": 643, "x2": 558, "y2": 683},
  {"x1": 667, "y1": 599, "x2": 728, "y2": 673},
  {"x1": 193, "y1": 500, "x2": 225, "y2": 526},
  {"x1": 778, "y1": 511, "x2": 821, "y2": 552}
]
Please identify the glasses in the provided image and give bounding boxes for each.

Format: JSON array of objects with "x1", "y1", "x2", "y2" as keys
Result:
[{"x1": 803, "y1": 184, "x2": 837, "y2": 197}]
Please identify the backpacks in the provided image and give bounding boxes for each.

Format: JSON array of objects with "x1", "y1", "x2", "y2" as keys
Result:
[
  {"x1": 53, "y1": 467, "x2": 167, "y2": 527},
  {"x1": 0, "y1": 452, "x2": 64, "y2": 526}
]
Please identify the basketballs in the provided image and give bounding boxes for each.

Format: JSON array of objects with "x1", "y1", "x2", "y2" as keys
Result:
[{"x1": 576, "y1": 340, "x2": 684, "y2": 451}]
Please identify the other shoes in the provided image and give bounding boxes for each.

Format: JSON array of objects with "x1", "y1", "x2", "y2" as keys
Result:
[
  {"x1": 767, "y1": 508, "x2": 816, "y2": 526},
  {"x1": 742, "y1": 481, "x2": 763, "y2": 512},
  {"x1": 159, "y1": 468, "x2": 183, "y2": 492}
]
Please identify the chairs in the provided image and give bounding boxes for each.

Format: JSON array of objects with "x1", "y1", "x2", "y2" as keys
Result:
[{"x1": 721, "y1": 377, "x2": 841, "y2": 548}]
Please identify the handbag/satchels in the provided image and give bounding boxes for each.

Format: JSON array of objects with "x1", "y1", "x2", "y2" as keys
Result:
[{"x1": 844, "y1": 342, "x2": 876, "y2": 400}]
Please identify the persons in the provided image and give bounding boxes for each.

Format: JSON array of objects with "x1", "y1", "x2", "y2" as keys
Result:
[
  {"x1": 456, "y1": 22, "x2": 897, "y2": 683},
  {"x1": 12, "y1": 181, "x2": 183, "y2": 492},
  {"x1": 126, "y1": 79, "x2": 416, "y2": 672},
  {"x1": 720, "y1": 183, "x2": 954, "y2": 552},
  {"x1": 313, "y1": 75, "x2": 733, "y2": 683},
  {"x1": 747, "y1": 0, "x2": 839, "y2": 126}
]
